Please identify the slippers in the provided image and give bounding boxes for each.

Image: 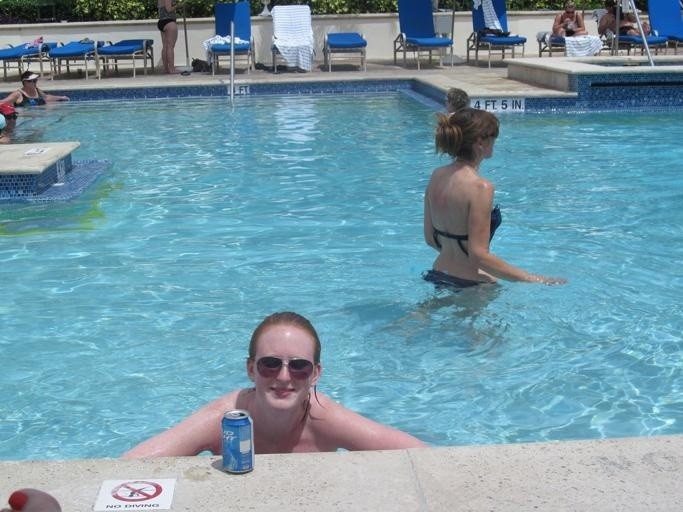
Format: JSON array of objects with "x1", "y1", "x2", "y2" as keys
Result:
[{"x1": 181, "y1": 71, "x2": 190, "y2": 76}]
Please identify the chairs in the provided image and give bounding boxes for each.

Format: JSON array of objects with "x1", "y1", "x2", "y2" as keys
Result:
[
  {"x1": 536, "y1": 32, "x2": 601, "y2": 57},
  {"x1": 211, "y1": 1, "x2": 254, "y2": 75},
  {"x1": 324, "y1": 32, "x2": 367, "y2": 73},
  {"x1": 467, "y1": 0, "x2": 527, "y2": 70},
  {"x1": 272, "y1": 5, "x2": 314, "y2": 73},
  {"x1": 96, "y1": 39, "x2": 154, "y2": 80},
  {"x1": 0, "y1": 42, "x2": 64, "y2": 80},
  {"x1": 646, "y1": 0, "x2": 683, "y2": 56},
  {"x1": 49, "y1": 40, "x2": 113, "y2": 81},
  {"x1": 393, "y1": 0, "x2": 453, "y2": 70},
  {"x1": 594, "y1": 8, "x2": 668, "y2": 56}
]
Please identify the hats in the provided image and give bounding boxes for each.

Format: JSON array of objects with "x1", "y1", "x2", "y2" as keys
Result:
[
  {"x1": 0, "y1": 103, "x2": 19, "y2": 115},
  {"x1": 21, "y1": 73, "x2": 39, "y2": 80},
  {"x1": 605, "y1": 0, "x2": 615, "y2": 6},
  {"x1": 564, "y1": 1, "x2": 576, "y2": 8}
]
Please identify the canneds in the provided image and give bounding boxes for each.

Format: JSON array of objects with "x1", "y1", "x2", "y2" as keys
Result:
[{"x1": 220, "y1": 409, "x2": 256, "y2": 474}]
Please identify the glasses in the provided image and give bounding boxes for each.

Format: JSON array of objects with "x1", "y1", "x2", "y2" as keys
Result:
[
  {"x1": 5, "y1": 115, "x2": 18, "y2": 119},
  {"x1": 4, "y1": 488, "x2": 61, "y2": 511},
  {"x1": 25, "y1": 79, "x2": 37, "y2": 82},
  {"x1": 252, "y1": 355, "x2": 316, "y2": 381},
  {"x1": 566, "y1": 11, "x2": 575, "y2": 14}
]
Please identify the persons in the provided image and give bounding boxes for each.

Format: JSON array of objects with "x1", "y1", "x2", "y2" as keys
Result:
[
  {"x1": 120, "y1": 312, "x2": 427, "y2": 458},
  {"x1": 422, "y1": 109, "x2": 568, "y2": 305},
  {"x1": 598, "y1": 0, "x2": 651, "y2": 37},
  {"x1": 445, "y1": 88, "x2": 468, "y2": 119},
  {"x1": 553, "y1": 0, "x2": 588, "y2": 37},
  {"x1": 157, "y1": 1, "x2": 182, "y2": 74},
  {"x1": 0, "y1": 72, "x2": 71, "y2": 106},
  {"x1": 0, "y1": 104, "x2": 20, "y2": 143}
]
